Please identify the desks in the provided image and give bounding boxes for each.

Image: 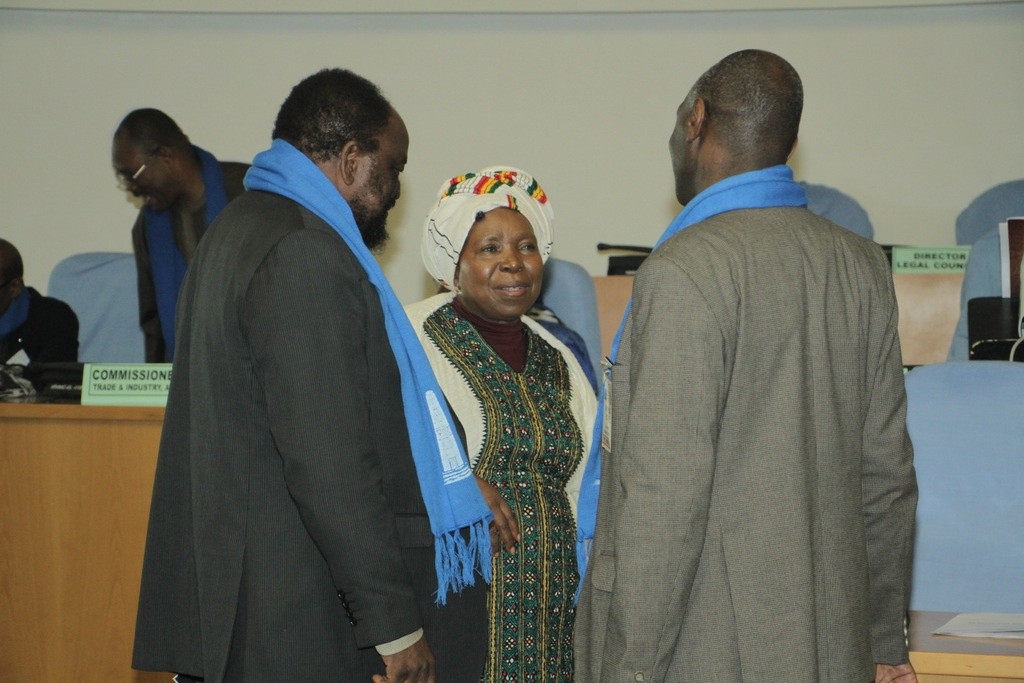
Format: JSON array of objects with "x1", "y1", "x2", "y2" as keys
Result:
[{"x1": 0, "y1": 274, "x2": 1024, "y2": 683}]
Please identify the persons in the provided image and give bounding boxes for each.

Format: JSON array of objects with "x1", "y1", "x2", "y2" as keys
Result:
[
  {"x1": 111, "y1": 108, "x2": 252, "y2": 363},
  {"x1": 946, "y1": 216, "x2": 1024, "y2": 361},
  {"x1": 402, "y1": 165, "x2": 600, "y2": 683},
  {"x1": 574, "y1": 48, "x2": 920, "y2": 683},
  {"x1": 130, "y1": 69, "x2": 494, "y2": 683},
  {"x1": 0, "y1": 239, "x2": 80, "y2": 401}
]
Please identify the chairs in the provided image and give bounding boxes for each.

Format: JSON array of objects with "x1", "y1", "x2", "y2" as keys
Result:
[
  {"x1": 793, "y1": 180, "x2": 1024, "y2": 616},
  {"x1": 48, "y1": 254, "x2": 144, "y2": 364}
]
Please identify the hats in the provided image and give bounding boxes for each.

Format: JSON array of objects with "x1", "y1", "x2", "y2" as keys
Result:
[{"x1": 421, "y1": 166, "x2": 554, "y2": 291}]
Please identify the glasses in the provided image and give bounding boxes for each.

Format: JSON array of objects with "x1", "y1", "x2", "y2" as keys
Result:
[{"x1": 117, "y1": 143, "x2": 170, "y2": 191}]
